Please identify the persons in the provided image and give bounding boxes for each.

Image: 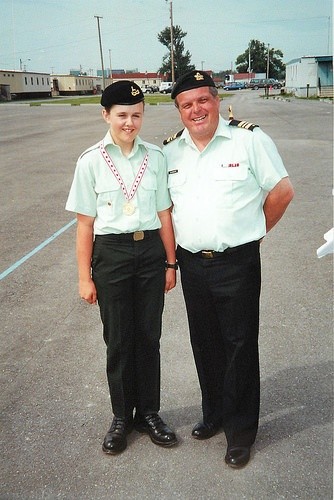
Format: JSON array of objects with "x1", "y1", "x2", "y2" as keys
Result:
[
  {"x1": 161, "y1": 70, "x2": 295, "y2": 469},
  {"x1": 65, "y1": 80, "x2": 178, "y2": 454}
]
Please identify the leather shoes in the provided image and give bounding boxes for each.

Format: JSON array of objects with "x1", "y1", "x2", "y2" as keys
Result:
[
  {"x1": 192, "y1": 418, "x2": 223, "y2": 439},
  {"x1": 132, "y1": 412, "x2": 178, "y2": 448},
  {"x1": 102, "y1": 415, "x2": 133, "y2": 454},
  {"x1": 225, "y1": 445, "x2": 250, "y2": 467}
]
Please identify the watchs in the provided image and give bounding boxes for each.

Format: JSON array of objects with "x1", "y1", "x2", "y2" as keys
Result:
[{"x1": 166, "y1": 262, "x2": 178, "y2": 271}]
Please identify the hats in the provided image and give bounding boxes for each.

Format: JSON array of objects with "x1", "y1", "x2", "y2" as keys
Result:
[
  {"x1": 171, "y1": 71, "x2": 215, "y2": 99},
  {"x1": 100, "y1": 81, "x2": 145, "y2": 107}
]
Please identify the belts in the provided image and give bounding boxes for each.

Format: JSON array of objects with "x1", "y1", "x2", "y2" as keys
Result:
[
  {"x1": 177, "y1": 241, "x2": 260, "y2": 258},
  {"x1": 95, "y1": 230, "x2": 160, "y2": 241}
]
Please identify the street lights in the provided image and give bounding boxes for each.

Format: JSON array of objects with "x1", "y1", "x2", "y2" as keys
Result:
[
  {"x1": 164, "y1": 0, "x2": 174, "y2": 87},
  {"x1": 94, "y1": 16, "x2": 106, "y2": 92},
  {"x1": 19, "y1": 58, "x2": 31, "y2": 71}
]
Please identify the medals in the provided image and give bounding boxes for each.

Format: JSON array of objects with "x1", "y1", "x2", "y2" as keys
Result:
[{"x1": 123, "y1": 202, "x2": 136, "y2": 215}]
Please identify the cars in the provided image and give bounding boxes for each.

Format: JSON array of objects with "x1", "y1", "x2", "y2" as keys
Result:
[
  {"x1": 140, "y1": 82, "x2": 177, "y2": 94},
  {"x1": 214, "y1": 79, "x2": 285, "y2": 91}
]
